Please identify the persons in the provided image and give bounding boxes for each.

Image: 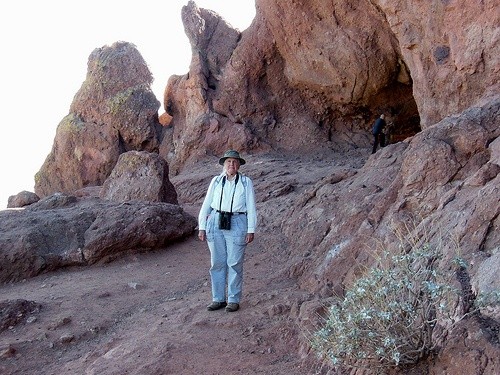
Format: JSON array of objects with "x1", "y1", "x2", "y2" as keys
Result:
[
  {"x1": 197, "y1": 150, "x2": 257, "y2": 312},
  {"x1": 371, "y1": 114, "x2": 387, "y2": 154}
]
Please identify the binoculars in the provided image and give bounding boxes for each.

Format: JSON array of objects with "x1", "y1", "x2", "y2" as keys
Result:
[{"x1": 218, "y1": 211, "x2": 231, "y2": 230}]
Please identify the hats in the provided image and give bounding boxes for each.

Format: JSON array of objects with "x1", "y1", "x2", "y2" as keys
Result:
[{"x1": 218, "y1": 150, "x2": 246, "y2": 165}]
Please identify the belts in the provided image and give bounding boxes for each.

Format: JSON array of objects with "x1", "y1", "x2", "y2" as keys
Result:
[{"x1": 212, "y1": 208, "x2": 245, "y2": 215}]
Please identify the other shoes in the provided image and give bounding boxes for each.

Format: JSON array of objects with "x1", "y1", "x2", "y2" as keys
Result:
[
  {"x1": 206, "y1": 302, "x2": 227, "y2": 311},
  {"x1": 225, "y1": 303, "x2": 239, "y2": 312}
]
